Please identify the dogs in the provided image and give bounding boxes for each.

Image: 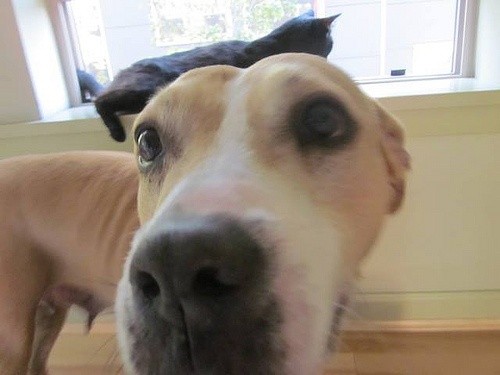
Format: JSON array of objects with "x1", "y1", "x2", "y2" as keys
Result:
[{"x1": 0, "y1": 52, "x2": 410, "y2": 375}]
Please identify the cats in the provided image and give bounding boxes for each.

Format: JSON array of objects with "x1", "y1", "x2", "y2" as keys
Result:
[{"x1": 76, "y1": 10, "x2": 342, "y2": 142}]
